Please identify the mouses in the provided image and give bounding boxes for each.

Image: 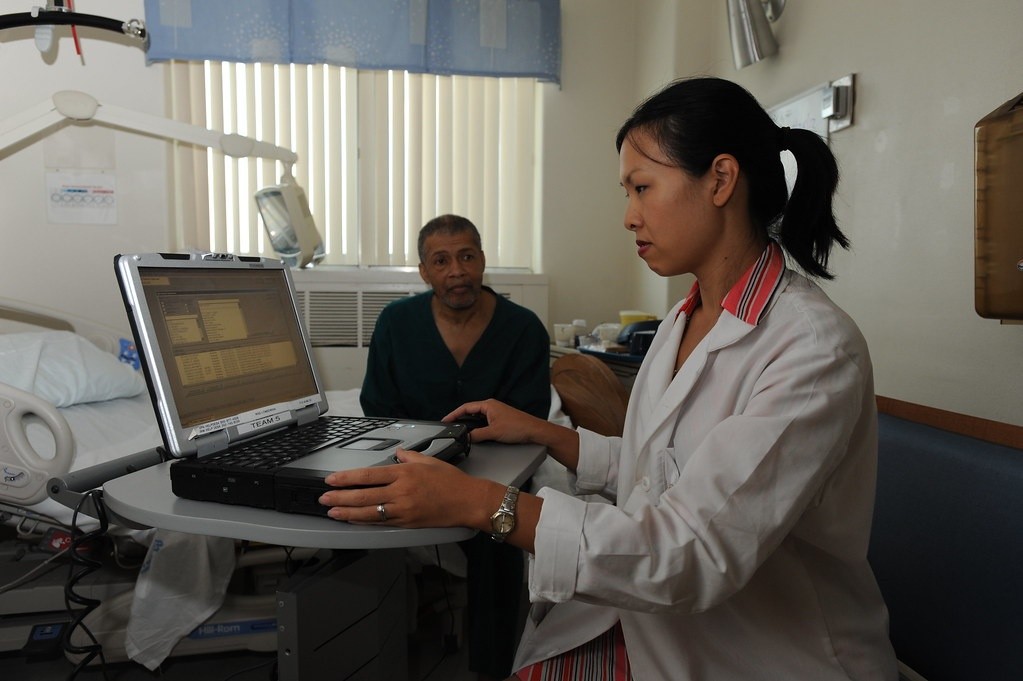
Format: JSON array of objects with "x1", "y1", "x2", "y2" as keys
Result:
[{"x1": 448, "y1": 413, "x2": 489, "y2": 432}]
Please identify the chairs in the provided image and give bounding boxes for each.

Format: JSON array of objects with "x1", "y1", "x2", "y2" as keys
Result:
[{"x1": 552, "y1": 352, "x2": 628, "y2": 437}]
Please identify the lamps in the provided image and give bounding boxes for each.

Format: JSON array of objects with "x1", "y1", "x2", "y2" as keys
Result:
[
  {"x1": 0, "y1": 90, "x2": 327, "y2": 269},
  {"x1": 726, "y1": 0, "x2": 786, "y2": 71}
]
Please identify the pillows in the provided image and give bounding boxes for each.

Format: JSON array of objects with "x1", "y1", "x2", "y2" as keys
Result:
[{"x1": 0, "y1": 331, "x2": 144, "y2": 409}]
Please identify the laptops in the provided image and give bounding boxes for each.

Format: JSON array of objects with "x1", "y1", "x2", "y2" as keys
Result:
[{"x1": 114, "y1": 252, "x2": 471, "y2": 519}]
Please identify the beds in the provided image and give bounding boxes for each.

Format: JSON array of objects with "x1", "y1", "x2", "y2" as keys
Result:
[{"x1": 0, "y1": 299, "x2": 297, "y2": 681}]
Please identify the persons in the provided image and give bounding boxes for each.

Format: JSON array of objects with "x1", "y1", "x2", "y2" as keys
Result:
[
  {"x1": 360, "y1": 214, "x2": 552, "y2": 681},
  {"x1": 318, "y1": 77, "x2": 901, "y2": 681}
]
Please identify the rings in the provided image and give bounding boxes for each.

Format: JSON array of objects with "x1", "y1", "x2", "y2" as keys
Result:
[{"x1": 376, "y1": 504, "x2": 387, "y2": 521}]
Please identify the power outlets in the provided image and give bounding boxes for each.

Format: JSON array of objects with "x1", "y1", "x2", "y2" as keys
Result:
[{"x1": 827, "y1": 73, "x2": 853, "y2": 132}]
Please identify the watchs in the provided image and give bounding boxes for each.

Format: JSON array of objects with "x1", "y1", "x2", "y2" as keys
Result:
[{"x1": 489, "y1": 486, "x2": 519, "y2": 544}]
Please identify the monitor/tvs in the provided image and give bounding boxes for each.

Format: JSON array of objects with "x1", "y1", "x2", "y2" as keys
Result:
[{"x1": 255, "y1": 186, "x2": 325, "y2": 267}]
